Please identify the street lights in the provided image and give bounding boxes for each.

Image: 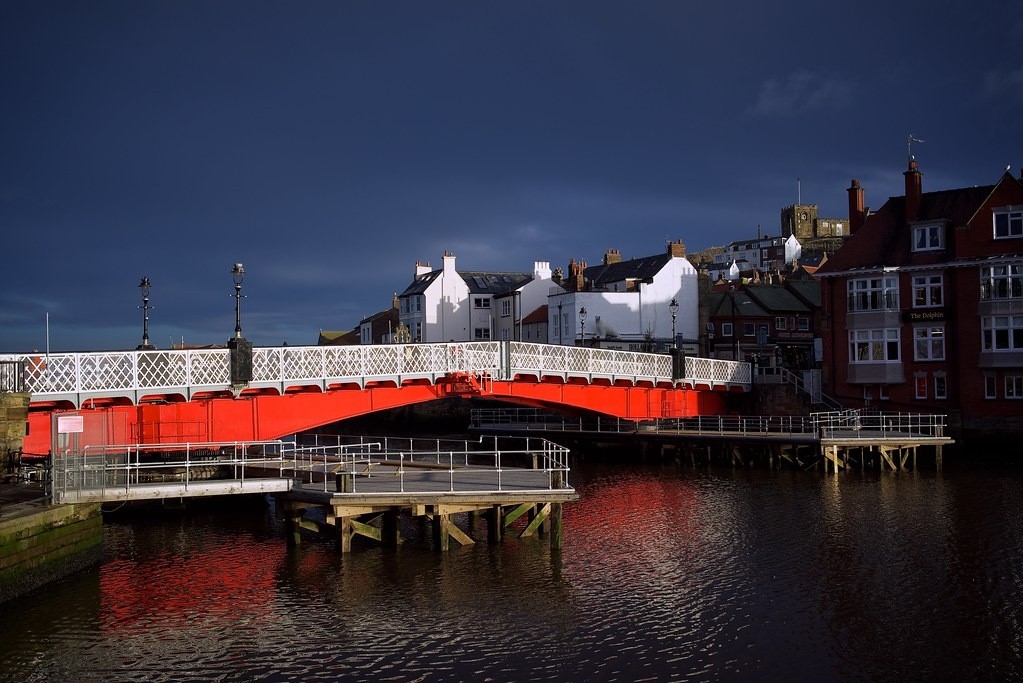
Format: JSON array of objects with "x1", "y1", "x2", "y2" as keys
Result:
[
  {"x1": 578, "y1": 306, "x2": 587, "y2": 347},
  {"x1": 137, "y1": 275, "x2": 155, "y2": 346},
  {"x1": 229, "y1": 263, "x2": 248, "y2": 338},
  {"x1": 669, "y1": 298, "x2": 679, "y2": 349}
]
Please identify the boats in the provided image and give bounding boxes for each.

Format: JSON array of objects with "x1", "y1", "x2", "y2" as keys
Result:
[{"x1": 12, "y1": 457, "x2": 52, "y2": 488}]
